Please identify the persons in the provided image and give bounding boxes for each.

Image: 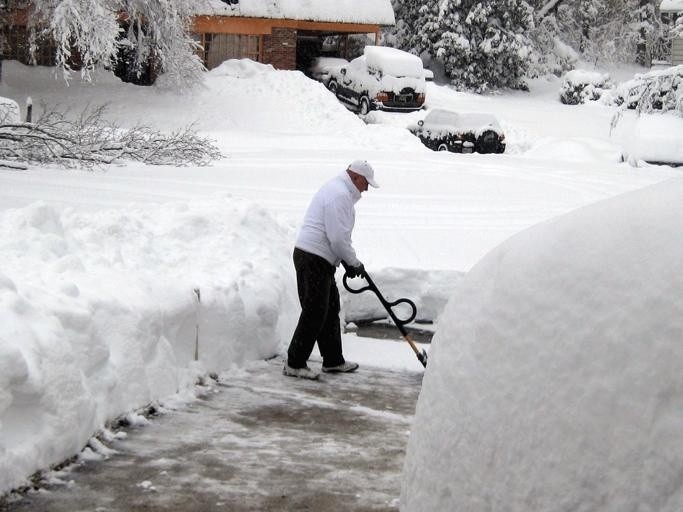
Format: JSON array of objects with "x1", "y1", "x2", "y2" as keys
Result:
[{"x1": 279, "y1": 156, "x2": 385, "y2": 380}]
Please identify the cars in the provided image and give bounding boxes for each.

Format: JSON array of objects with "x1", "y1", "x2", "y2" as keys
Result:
[{"x1": 305, "y1": 55, "x2": 348, "y2": 85}]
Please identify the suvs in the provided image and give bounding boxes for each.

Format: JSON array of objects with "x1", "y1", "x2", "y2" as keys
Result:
[
  {"x1": 407, "y1": 108, "x2": 512, "y2": 157},
  {"x1": 323, "y1": 44, "x2": 428, "y2": 116}
]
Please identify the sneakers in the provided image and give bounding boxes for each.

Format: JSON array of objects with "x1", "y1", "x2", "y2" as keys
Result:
[
  {"x1": 322, "y1": 362, "x2": 359, "y2": 372},
  {"x1": 283, "y1": 361, "x2": 317, "y2": 379}
]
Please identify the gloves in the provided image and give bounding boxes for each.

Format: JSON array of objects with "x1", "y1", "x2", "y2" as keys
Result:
[{"x1": 341, "y1": 260, "x2": 365, "y2": 278}]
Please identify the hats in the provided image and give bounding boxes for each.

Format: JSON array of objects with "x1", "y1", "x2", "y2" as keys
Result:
[{"x1": 349, "y1": 160, "x2": 380, "y2": 188}]
